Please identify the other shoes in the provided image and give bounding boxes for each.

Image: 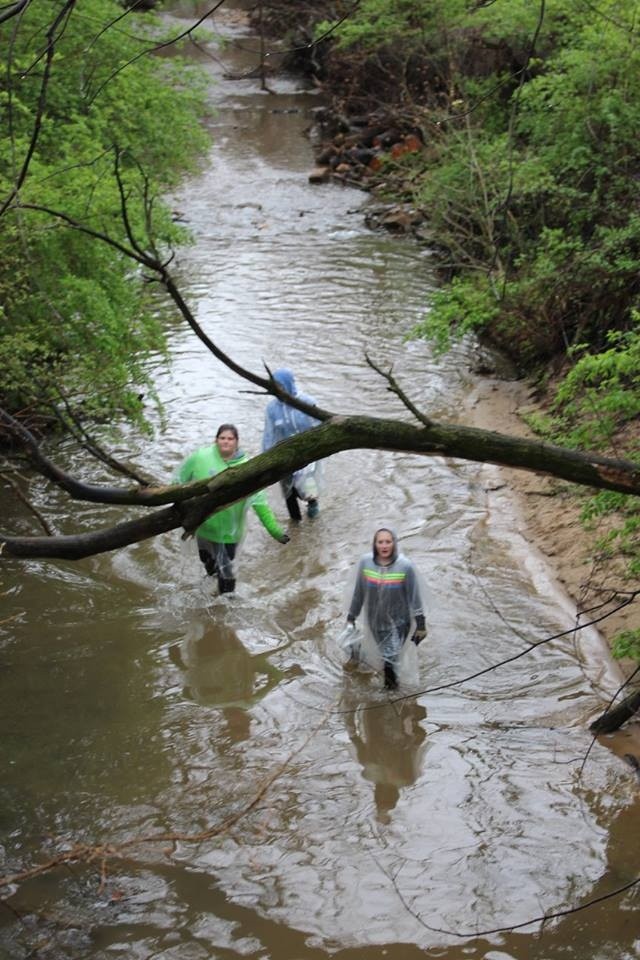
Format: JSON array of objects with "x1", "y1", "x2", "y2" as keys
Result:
[{"x1": 308, "y1": 499, "x2": 319, "y2": 519}]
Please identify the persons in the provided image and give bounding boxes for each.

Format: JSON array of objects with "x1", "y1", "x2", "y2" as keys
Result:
[
  {"x1": 262, "y1": 368, "x2": 318, "y2": 521},
  {"x1": 347, "y1": 527, "x2": 428, "y2": 693},
  {"x1": 171, "y1": 424, "x2": 292, "y2": 596}
]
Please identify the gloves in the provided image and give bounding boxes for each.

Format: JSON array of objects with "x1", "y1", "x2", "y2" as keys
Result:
[
  {"x1": 181, "y1": 531, "x2": 195, "y2": 541},
  {"x1": 411, "y1": 630, "x2": 427, "y2": 646},
  {"x1": 346, "y1": 614, "x2": 356, "y2": 623},
  {"x1": 280, "y1": 534, "x2": 290, "y2": 544}
]
